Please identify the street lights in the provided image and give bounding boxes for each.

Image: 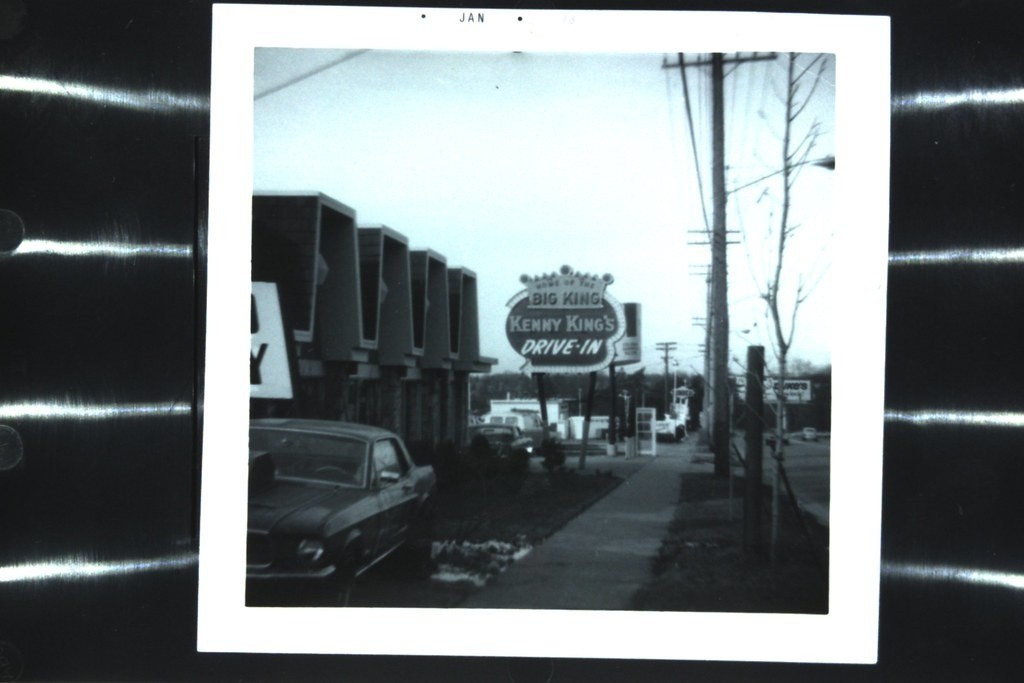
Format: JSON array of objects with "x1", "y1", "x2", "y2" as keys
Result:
[{"x1": 707, "y1": 155, "x2": 835, "y2": 503}]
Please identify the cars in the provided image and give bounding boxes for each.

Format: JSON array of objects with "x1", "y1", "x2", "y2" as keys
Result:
[
  {"x1": 469, "y1": 423, "x2": 535, "y2": 469},
  {"x1": 244, "y1": 417, "x2": 441, "y2": 607}
]
